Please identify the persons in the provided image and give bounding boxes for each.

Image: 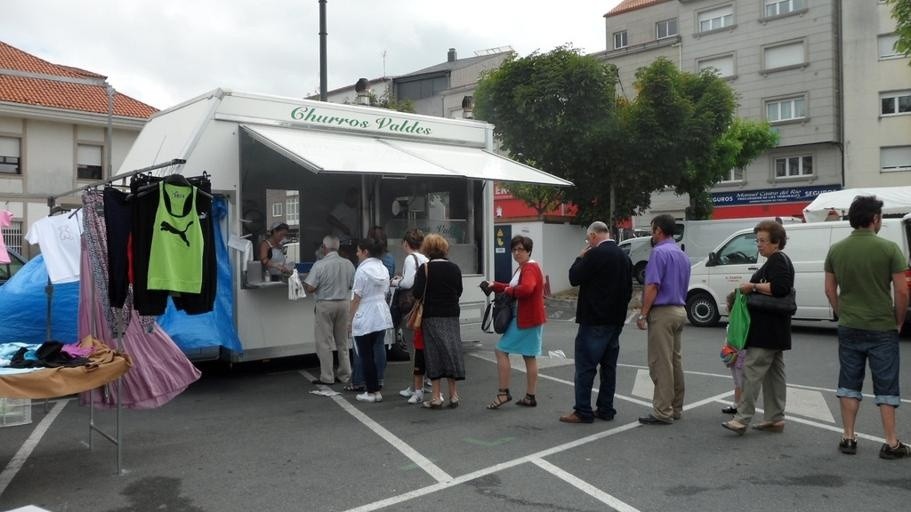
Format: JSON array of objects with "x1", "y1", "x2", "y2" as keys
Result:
[
  {"x1": 721, "y1": 222, "x2": 794, "y2": 436},
  {"x1": 390, "y1": 227, "x2": 430, "y2": 405},
  {"x1": 477, "y1": 235, "x2": 547, "y2": 410},
  {"x1": 636, "y1": 214, "x2": 691, "y2": 425},
  {"x1": 720, "y1": 291, "x2": 747, "y2": 415},
  {"x1": 412, "y1": 232, "x2": 465, "y2": 410},
  {"x1": 342, "y1": 226, "x2": 395, "y2": 391},
  {"x1": 258, "y1": 220, "x2": 290, "y2": 281},
  {"x1": 824, "y1": 194, "x2": 911, "y2": 459},
  {"x1": 346, "y1": 235, "x2": 394, "y2": 403},
  {"x1": 302, "y1": 236, "x2": 356, "y2": 387},
  {"x1": 559, "y1": 221, "x2": 633, "y2": 424}
]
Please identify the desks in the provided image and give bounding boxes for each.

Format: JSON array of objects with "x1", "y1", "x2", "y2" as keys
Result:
[{"x1": 0, "y1": 349, "x2": 126, "y2": 476}]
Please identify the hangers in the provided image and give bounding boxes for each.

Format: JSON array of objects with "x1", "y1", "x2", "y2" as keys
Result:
[{"x1": 46, "y1": 160, "x2": 213, "y2": 217}]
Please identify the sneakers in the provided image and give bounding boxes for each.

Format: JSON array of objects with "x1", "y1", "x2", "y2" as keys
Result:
[
  {"x1": 879, "y1": 441, "x2": 911, "y2": 459},
  {"x1": 840, "y1": 438, "x2": 857, "y2": 453},
  {"x1": 312, "y1": 377, "x2": 382, "y2": 403},
  {"x1": 400, "y1": 382, "x2": 458, "y2": 409}
]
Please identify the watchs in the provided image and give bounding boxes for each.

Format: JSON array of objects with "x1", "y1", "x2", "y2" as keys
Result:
[
  {"x1": 752, "y1": 282, "x2": 757, "y2": 292},
  {"x1": 637, "y1": 311, "x2": 648, "y2": 319}
]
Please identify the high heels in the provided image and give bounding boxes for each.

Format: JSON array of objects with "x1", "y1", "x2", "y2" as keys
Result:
[
  {"x1": 722, "y1": 419, "x2": 747, "y2": 437},
  {"x1": 752, "y1": 419, "x2": 785, "y2": 433}
]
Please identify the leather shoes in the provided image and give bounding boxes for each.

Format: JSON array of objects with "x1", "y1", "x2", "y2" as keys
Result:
[
  {"x1": 639, "y1": 415, "x2": 663, "y2": 423},
  {"x1": 560, "y1": 411, "x2": 582, "y2": 423},
  {"x1": 722, "y1": 406, "x2": 737, "y2": 413}
]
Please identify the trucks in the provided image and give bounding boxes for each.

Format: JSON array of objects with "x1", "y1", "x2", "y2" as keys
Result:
[{"x1": 630, "y1": 218, "x2": 806, "y2": 288}]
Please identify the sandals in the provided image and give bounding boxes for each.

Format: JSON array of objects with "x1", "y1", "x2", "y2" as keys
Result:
[
  {"x1": 516, "y1": 394, "x2": 537, "y2": 406},
  {"x1": 487, "y1": 388, "x2": 512, "y2": 409}
]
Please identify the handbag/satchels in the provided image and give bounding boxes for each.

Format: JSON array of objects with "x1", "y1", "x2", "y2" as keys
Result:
[
  {"x1": 493, "y1": 292, "x2": 512, "y2": 333},
  {"x1": 396, "y1": 287, "x2": 423, "y2": 328},
  {"x1": 727, "y1": 288, "x2": 749, "y2": 353},
  {"x1": 748, "y1": 283, "x2": 798, "y2": 316}
]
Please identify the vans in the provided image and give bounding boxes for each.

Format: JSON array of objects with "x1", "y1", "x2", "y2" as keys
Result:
[
  {"x1": 676, "y1": 212, "x2": 910, "y2": 335},
  {"x1": 618, "y1": 233, "x2": 681, "y2": 258}
]
